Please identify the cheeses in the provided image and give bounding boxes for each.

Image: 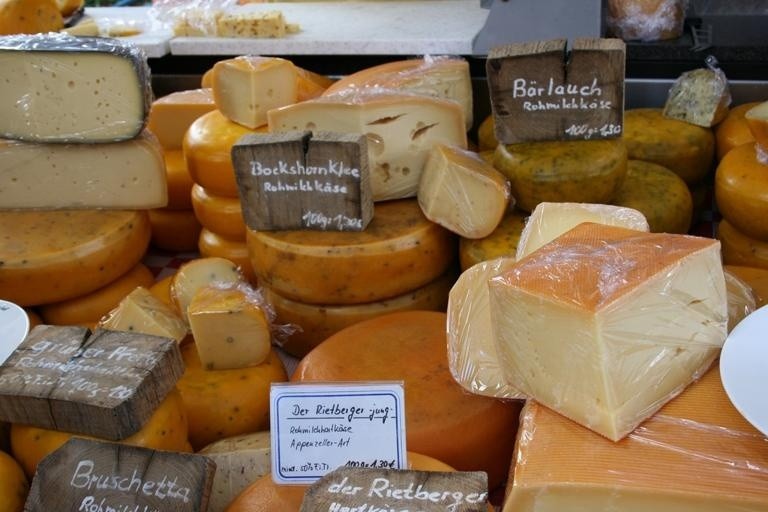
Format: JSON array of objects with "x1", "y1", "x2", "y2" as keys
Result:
[{"x1": 0, "y1": 0, "x2": 768, "y2": 512}]
